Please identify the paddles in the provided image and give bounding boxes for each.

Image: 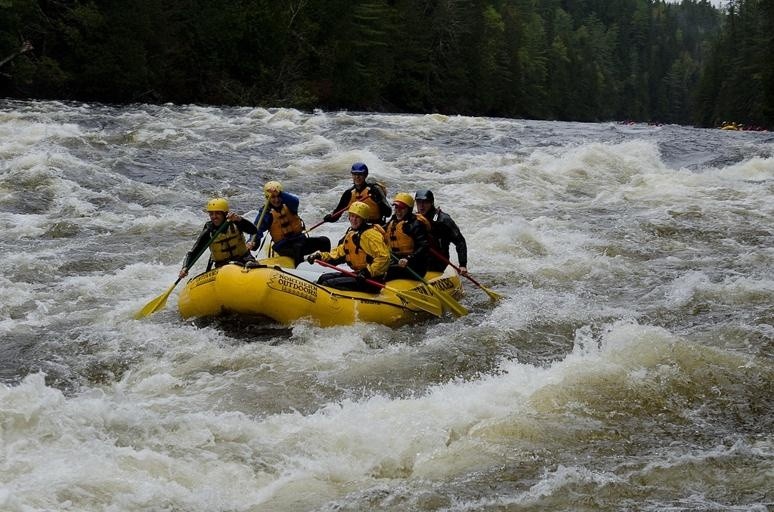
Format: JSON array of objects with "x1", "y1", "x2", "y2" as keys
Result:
[
  {"x1": 134, "y1": 217, "x2": 231, "y2": 320},
  {"x1": 390, "y1": 253, "x2": 468, "y2": 317},
  {"x1": 312, "y1": 260, "x2": 442, "y2": 318},
  {"x1": 429, "y1": 248, "x2": 512, "y2": 302},
  {"x1": 263, "y1": 194, "x2": 371, "y2": 258}
]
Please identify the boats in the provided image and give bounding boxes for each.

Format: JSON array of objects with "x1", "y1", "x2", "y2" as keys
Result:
[{"x1": 178, "y1": 245, "x2": 463, "y2": 334}]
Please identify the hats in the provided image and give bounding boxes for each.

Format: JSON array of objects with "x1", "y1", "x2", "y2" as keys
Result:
[{"x1": 392, "y1": 202, "x2": 407, "y2": 209}]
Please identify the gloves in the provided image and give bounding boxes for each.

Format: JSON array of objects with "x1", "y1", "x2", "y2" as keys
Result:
[
  {"x1": 307, "y1": 250, "x2": 321, "y2": 265},
  {"x1": 323, "y1": 214, "x2": 332, "y2": 222},
  {"x1": 356, "y1": 268, "x2": 370, "y2": 282}
]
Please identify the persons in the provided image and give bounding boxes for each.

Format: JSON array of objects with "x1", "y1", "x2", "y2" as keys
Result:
[
  {"x1": 178, "y1": 198, "x2": 258, "y2": 281},
  {"x1": 381, "y1": 193, "x2": 433, "y2": 282},
  {"x1": 246, "y1": 181, "x2": 331, "y2": 270},
  {"x1": 412, "y1": 189, "x2": 467, "y2": 277},
  {"x1": 322, "y1": 162, "x2": 391, "y2": 247},
  {"x1": 307, "y1": 201, "x2": 391, "y2": 295}
]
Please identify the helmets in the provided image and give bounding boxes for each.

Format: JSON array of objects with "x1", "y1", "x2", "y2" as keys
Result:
[
  {"x1": 415, "y1": 190, "x2": 433, "y2": 200},
  {"x1": 393, "y1": 192, "x2": 414, "y2": 207},
  {"x1": 348, "y1": 201, "x2": 370, "y2": 219},
  {"x1": 263, "y1": 180, "x2": 283, "y2": 197},
  {"x1": 350, "y1": 163, "x2": 368, "y2": 176},
  {"x1": 207, "y1": 198, "x2": 229, "y2": 213}
]
